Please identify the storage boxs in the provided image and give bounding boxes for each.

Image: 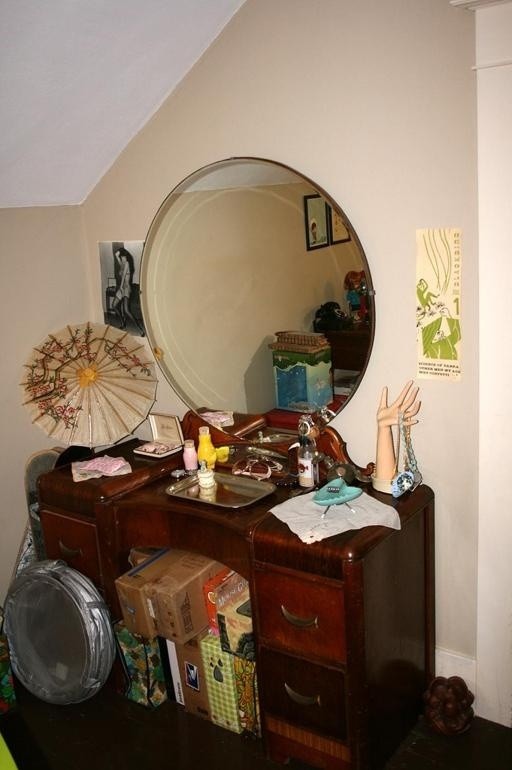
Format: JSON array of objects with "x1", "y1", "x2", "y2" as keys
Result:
[{"x1": 111, "y1": 545, "x2": 254, "y2": 739}]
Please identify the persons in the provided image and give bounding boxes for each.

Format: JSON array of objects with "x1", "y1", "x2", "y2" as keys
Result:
[
  {"x1": 111, "y1": 247, "x2": 144, "y2": 337},
  {"x1": 372, "y1": 379, "x2": 421, "y2": 482}
]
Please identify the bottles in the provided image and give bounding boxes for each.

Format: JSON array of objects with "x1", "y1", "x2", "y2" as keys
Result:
[
  {"x1": 180, "y1": 425, "x2": 216, "y2": 470},
  {"x1": 296, "y1": 435, "x2": 320, "y2": 488}
]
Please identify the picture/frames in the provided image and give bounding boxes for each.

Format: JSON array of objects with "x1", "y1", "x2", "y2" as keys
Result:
[{"x1": 303, "y1": 193, "x2": 350, "y2": 251}]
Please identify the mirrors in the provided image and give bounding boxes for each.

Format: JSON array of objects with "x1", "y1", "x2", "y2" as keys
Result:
[{"x1": 136, "y1": 157, "x2": 375, "y2": 445}]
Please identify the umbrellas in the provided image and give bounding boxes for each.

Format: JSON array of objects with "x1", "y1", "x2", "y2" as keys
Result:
[{"x1": 17, "y1": 320, "x2": 160, "y2": 453}]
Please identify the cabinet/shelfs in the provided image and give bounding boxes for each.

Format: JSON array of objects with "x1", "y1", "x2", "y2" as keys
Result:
[{"x1": 36, "y1": 438, "x2": 438, "y2": 770}]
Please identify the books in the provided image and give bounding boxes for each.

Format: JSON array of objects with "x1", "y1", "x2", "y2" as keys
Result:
[{"x1": 267, "y1": 327, "x2": 330, "y2": 354}]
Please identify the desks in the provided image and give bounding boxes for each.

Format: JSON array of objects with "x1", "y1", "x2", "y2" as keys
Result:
[{"x1": 309, "y1": 328, "x2": 372, "y2": 373}]
